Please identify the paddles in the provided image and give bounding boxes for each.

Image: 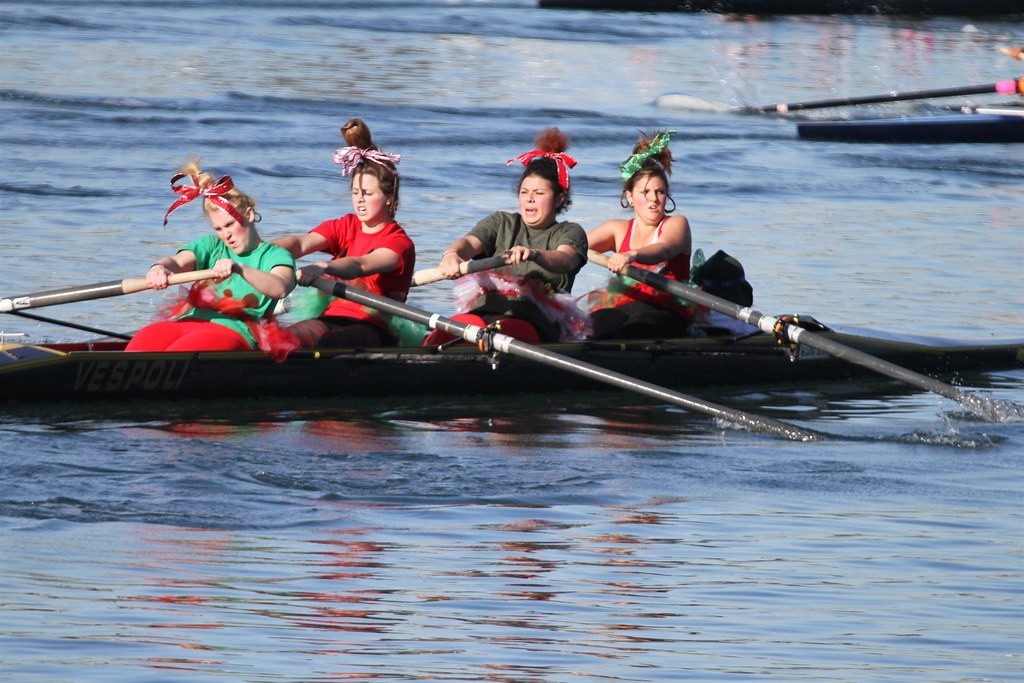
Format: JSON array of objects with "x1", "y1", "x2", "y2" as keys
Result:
[
  {"x1": 584, "y1": 246, "x2": 987, "y2": 412},
  {"x1": 294, "y1": 269, "x2": 825, "y2": 442},
  {"x1": 997, "y1": 46, "x2": 1024, "y2": 61},
  {"x1": 410, "y1": 252, "x2": 508, "y2": 288},
  {"x1": 649, "y1": 77, "x2": 1019, "y2": 115},
  {"x1": 0, "y1": 268, "x2": 220, "y2": 315}
]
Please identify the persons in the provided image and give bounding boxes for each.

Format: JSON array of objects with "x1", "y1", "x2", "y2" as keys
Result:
[
  {"x1": 587, "y1": 129, "x2": 700, "y2": 338},
  {"x1": 271, "y1": 118, "x2": 416, "y2": 351},
  {"x1": 125, "y1": 158, "x2": 298, "y2": 352},
  {"x1": 422, "y1": 128, "x2": 589, "y2": 347}
]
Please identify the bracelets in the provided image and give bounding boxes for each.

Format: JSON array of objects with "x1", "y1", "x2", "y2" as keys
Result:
[
  {"x1": 150, "y1": 263, "x2": 159, "y2": 269},
  {"x1": 443, "y1": 251, "x2": 461, "y2": 257}
]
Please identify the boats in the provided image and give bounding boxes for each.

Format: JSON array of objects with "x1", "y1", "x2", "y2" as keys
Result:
[
  {"x1": 0, "y1": 325, "x2": 1024, "y2": 406},
  {"x1": 790, "y1": 100, "x2": 1024, "y2": 142}
]
